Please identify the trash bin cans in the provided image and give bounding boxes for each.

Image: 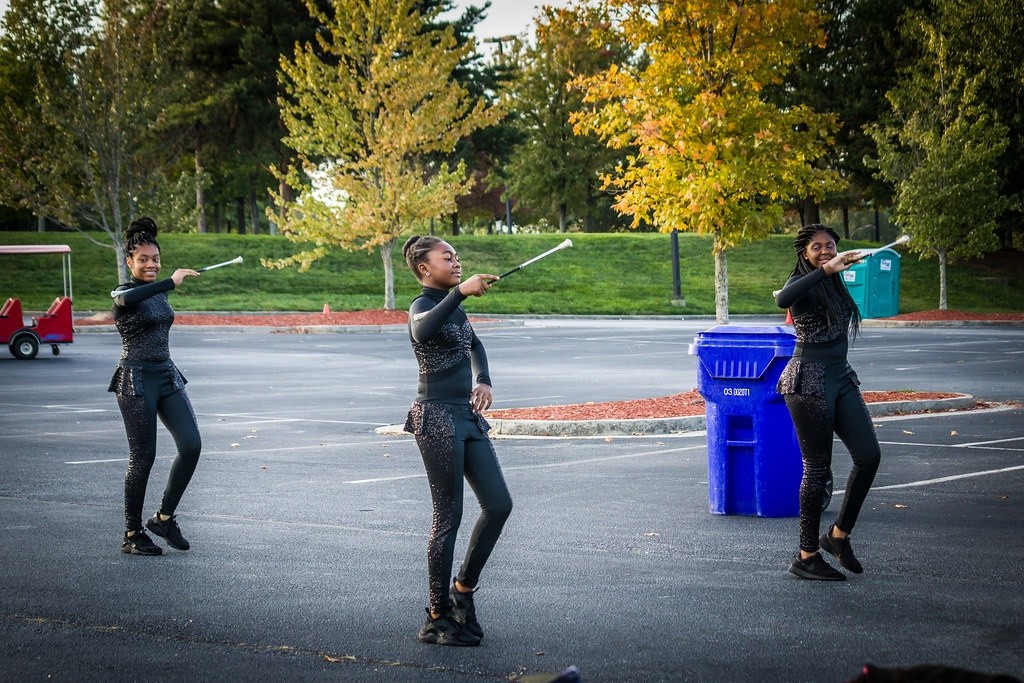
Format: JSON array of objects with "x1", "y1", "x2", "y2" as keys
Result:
[
  {"x1": 694, "y1": 322, "x2": 834, "y2": 520},
  {"x1": 836, "y1": 247, "x2": 902, "y2": 320}
]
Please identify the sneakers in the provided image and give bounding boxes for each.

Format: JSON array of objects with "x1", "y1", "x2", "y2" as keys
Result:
[
  {"x1": 418, "y1": 606, "x2": 481, "y2": 647},
  {"x1": 120, "y1": 527, "x2": 163, "y2": 555},
  {"x1": 144, "y1": 510, "x2": 190, "y2": 551},
  {"x1": 787, "y1": 550, "x2": 846, "y2": 581},
  {"x1": 817, "y1": 524, "x2": 864, "y2": 575},
  {"x1": 446, "y1": 576, "x2": 485, "y2": 639}
]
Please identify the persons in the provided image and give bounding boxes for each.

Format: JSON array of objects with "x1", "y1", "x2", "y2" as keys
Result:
[
  {"x1": 108, "y1": 217, "x2": 202, "y2": 553},
  {"x1": 402, "y1": 236, "x2": 512, "y2": 646},
  {"x1": 776, "y1": 225, "x2": 881, "y2": 581}
]
